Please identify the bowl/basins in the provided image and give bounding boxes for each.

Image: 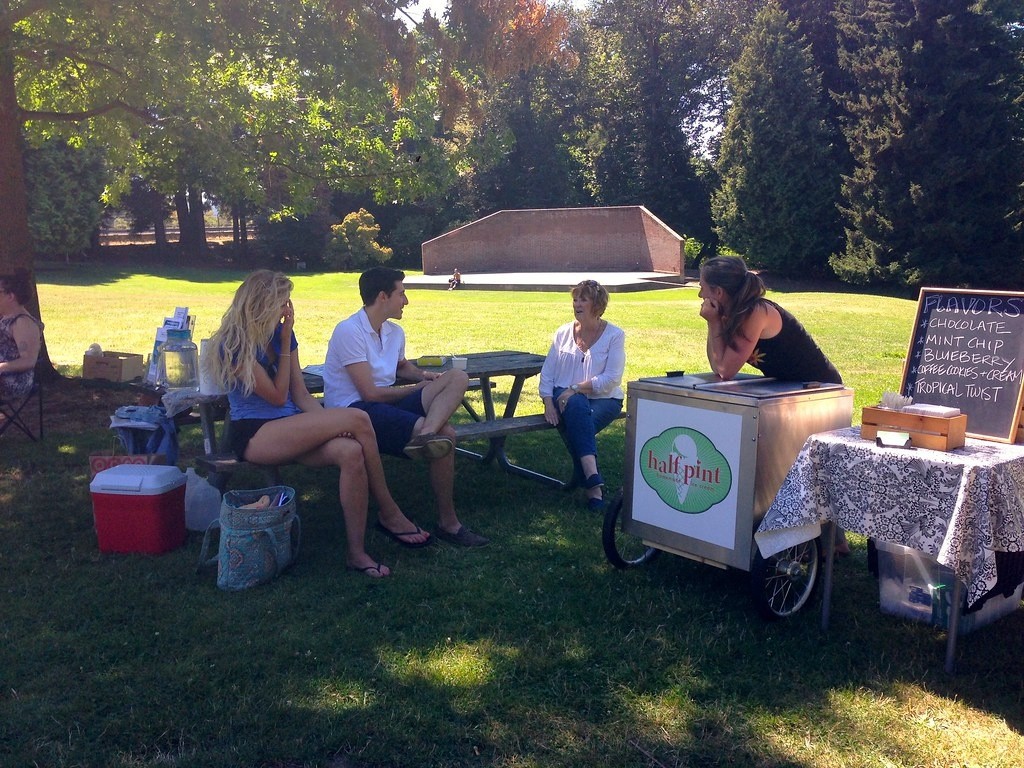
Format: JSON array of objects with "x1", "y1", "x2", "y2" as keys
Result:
[{"x1": 452, "y1": 358, "x2": 468, "y2": 370}]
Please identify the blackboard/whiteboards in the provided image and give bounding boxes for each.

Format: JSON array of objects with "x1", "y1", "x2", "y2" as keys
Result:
[{"x1": 899, "y1": 286, "x2": 1024, "y2": 444}]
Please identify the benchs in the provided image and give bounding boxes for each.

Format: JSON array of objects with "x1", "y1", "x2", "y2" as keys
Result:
[{"x1": 131, "y1": 379, "x2": 627, "y2": 491}]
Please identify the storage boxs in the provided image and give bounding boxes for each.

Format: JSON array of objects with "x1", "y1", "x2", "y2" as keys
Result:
[
  {"x1": 89, "y1": 449, "x2": 164, "y2": 482},
  {"x1": 83, "y1": 352, "x2": 142, "y2": 382},
  {"x1": 90, "y1": 463, "x2": 189, "y2": 553},
  {"x1": 861, "y1": 404, "x2": 967, "y2": 452},
  {"x1": 877, "y1": 539, "x2": 1021, "y2": 631}
]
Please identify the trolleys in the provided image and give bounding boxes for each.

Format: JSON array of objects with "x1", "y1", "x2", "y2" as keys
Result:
[{"x1": 601, "y1": 371, "x2": 855, "y2": 623}]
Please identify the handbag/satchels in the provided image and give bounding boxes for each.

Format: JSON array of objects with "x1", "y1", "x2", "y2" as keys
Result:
[
  {"x1": 90, "y1": 434, "x2": 167, "y2": 481},
  {"x1": 196, "y1": 485, "x2": 301, "y2": 592}
]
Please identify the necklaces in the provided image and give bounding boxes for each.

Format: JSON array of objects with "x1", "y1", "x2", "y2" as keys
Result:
[{"x1": 580, "y1": 321, "x2": 602, "y2": 353}]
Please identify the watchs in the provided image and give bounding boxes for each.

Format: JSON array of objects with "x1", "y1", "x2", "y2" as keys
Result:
[{"x1": 570, "y1": 384, "x2": 579, "y2": 393}]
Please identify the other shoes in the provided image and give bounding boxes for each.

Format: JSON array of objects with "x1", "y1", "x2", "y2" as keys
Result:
[
  {"x1": 435, "y1": 522, "x2": 489, "y2": 547},
  {"x1": 585, "y1": 474, "x2": 604, "y2": 490},
  {"x1": 403, "y1": 432, "x2": 454, "y2": 461},
  {"x1": 588, "y1": 494, "x2": 603, "y2": 511}
]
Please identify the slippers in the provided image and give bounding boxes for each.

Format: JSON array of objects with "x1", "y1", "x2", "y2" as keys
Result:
[
  {"x1": 375, "y1": 519, "x2": 433, "y2": 548},
  {"x1": 345, "y1": 562, "x2": 392, "y2": 578}
]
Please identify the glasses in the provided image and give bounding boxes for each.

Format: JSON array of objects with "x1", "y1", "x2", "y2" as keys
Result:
[{"x1": 578, "y1": 280, "x2": 599, "y2": 291}]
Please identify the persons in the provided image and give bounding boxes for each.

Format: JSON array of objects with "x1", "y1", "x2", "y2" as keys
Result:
[
  {"x1": 323, "y1": 266, "x2": 492, "y2": 547},
  {"x1": 202, "y1": 272, "x2": 434, "y2": 581},
  {"x1": 539, "y1": 281, "x2": 625, "y2": 510},
  {"x1": 698, "y1": 257, "x2": 842, "y2": 384},
  {"x1": 448, "y1": 268, "x2": 460, "y2": 290},
  {"x1": 0, "y1": 268, "x2": 42, "y2": 411}
]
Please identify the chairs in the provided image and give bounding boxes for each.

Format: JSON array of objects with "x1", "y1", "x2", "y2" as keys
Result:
[{"x1": 0, "y1": 336, "x2": 45, "y2": 442}]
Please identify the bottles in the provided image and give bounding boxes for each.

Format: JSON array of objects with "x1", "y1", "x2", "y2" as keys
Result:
[{"x1": 160, "y1": 329, "x2": 200, "y2": 392}]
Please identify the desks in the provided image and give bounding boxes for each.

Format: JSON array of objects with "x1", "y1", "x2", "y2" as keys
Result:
[
  {"x1": 755, "y1": 426, "x2": 1024, "y2": 672},
  {"x1": 170, "y1": 350, "x2": 547, "y2": 454}
]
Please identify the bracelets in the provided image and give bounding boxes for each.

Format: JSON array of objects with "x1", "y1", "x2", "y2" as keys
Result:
[{"x1": 280, "y1": 354, "x2": 290, "y2": 358}]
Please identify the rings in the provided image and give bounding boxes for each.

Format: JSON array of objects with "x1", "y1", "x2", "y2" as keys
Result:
[{"x1": 562, "y1": 400, "x2": 565, "y2": 405}]
